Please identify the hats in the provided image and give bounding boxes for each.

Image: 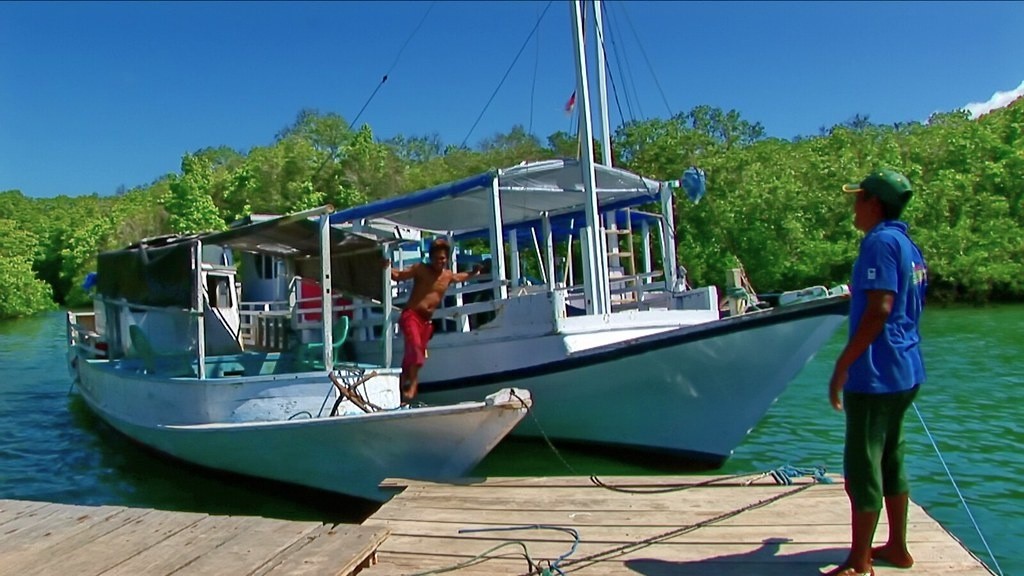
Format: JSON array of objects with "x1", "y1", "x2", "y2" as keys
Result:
[{"x1": 842, "y1": 169, "x2": 913, "y2": 208}]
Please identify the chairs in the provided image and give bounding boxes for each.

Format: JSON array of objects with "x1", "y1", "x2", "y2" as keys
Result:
[
  {"x1": 296, "y1": 315, "x2": 349, "y2": 371},
  {"x1": 129, "y1": 324, "x2": 195, "y2": 378}
]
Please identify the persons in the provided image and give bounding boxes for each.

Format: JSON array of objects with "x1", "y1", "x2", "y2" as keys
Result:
[
  {"x1": 818, "y1": 172, "x2": 927, "y2": 576},
  {"x1": 378, "y1": 239, "x2": 482, "y2": 404}
]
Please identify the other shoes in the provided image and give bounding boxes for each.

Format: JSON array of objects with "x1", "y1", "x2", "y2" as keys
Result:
[{"x1": 401, "y1": 384, "x2": 416, "y2": 407}]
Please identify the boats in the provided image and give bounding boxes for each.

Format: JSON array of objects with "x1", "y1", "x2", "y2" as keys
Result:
[{"x1": 65, "y1": 1, "x2": 850, "y2": 517}]
